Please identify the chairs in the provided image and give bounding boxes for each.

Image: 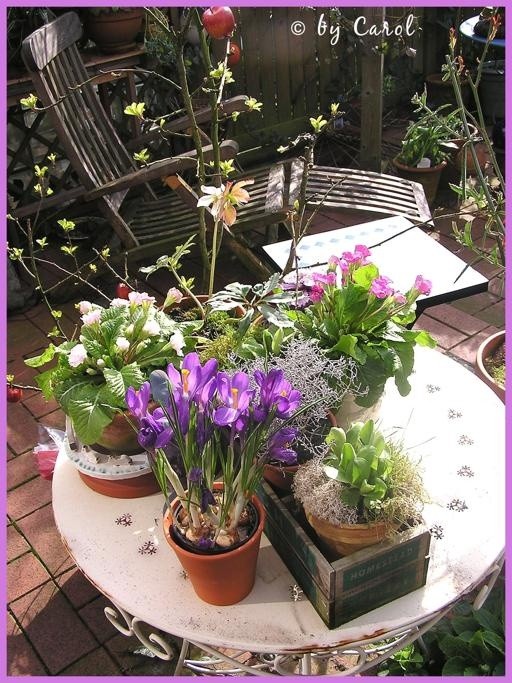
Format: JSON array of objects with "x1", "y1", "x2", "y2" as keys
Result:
[{"x1": 22, "y1": 11, "x2": 441, "y2": 302}]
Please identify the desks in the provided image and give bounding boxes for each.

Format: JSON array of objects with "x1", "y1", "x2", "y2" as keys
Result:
[{"x1": 51, "y1": 343, "x2": 504, "y2": 674}]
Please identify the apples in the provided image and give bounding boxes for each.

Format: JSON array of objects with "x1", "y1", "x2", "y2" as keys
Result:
[
  {"x1": 203, "y1": 5, "x2": 235, "y2": 39},
  {"x1": 7, "y1": 386, "x2": 22, "y2": 402},
  {"x1": 228, "y1": 44, "x2": 240, "y2": 67},
  {"x1": 117, "y1": 283, "x2": 131, "y2": 299}
]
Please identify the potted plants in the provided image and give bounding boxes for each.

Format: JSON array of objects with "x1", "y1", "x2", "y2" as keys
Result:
[{"x1": 391, "y1": 81, "x2": 478, "y2": 212}]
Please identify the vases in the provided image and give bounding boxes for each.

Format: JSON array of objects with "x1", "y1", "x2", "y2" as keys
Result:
[{"x1": 161, "y1": 480, "x2": 405, "y2": 606}]
